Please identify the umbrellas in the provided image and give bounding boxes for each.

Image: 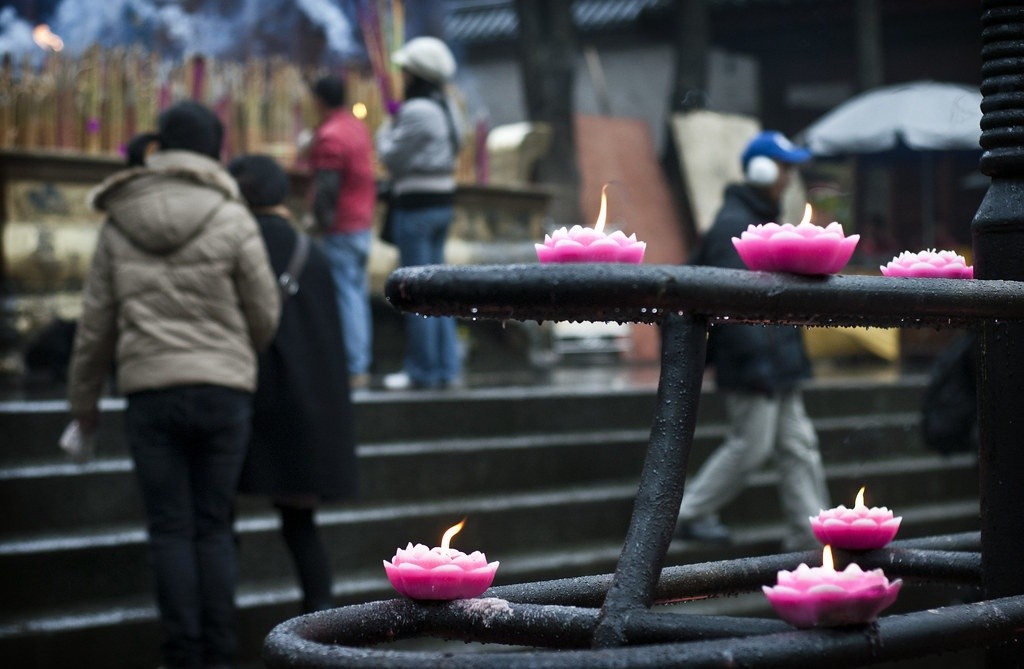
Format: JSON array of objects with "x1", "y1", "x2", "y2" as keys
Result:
[{"x1": 801, "y1": 82, "x2": 981, "y2": 163}]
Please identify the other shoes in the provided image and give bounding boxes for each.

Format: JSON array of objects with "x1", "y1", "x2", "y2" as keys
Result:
[
  {"x1": 347, "y1": 371, "x2": 371, "y2": 391},
  {"x1": 422, "y1": 374, "x2": 467, "y2": 388},
  {"x1": 676, "y1": 517, "x2": 731, "y2": 543},
  {"x1": 382, "y1": 370, "x2": 418, "y2": 390},
  {"x1": 378, "y1": 198, "x2": 396, "y2": 245}
]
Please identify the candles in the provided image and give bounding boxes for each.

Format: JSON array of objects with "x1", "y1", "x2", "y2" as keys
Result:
[
  {"x1": 762, "y1": 544, "x2": 902, "y2": 630},
  {"x1": 382, "y1": 519, "x2": 500, "y2": 602},
  {"x1": 731, "y1": 202, "x2": 860, "y2": 275},
  {"x1": 534, "y1": 184, "x2": 645, "y2": 265},
  {"x1": 880, "y1": 248, "x2": 973, "y2": 278},
  {"x1": 808, "y1": 486, "x2": 902, "y2": 548}
]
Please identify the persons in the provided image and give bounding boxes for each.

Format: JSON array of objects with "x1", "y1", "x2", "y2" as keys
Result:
[
  {"x1": 376, "y1": 38, "x2": 463, "y2": 390},
  {"x1": 232, "y1": 151, "x2": 352, "y2": 608},
  {"x1": 301, "y1": 68, "x2": 376, "y2": 386},
  {"x1": 66, "y1": 99, "x2": 279, "y2": 669},
  {"x1": 922, "y1": 328, "x2": 976, "y2": 451},
  {"x1": 681, "y1": 136, "x2": 830, "y2": 550}
]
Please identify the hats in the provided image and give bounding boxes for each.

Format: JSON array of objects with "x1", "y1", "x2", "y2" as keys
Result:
[
  {"x1": 390, "y1": 36, "x2": 455, "y2": 84},
  {"x1": 743, "y1": 132, "x2": 814, "y2": 170}
]
